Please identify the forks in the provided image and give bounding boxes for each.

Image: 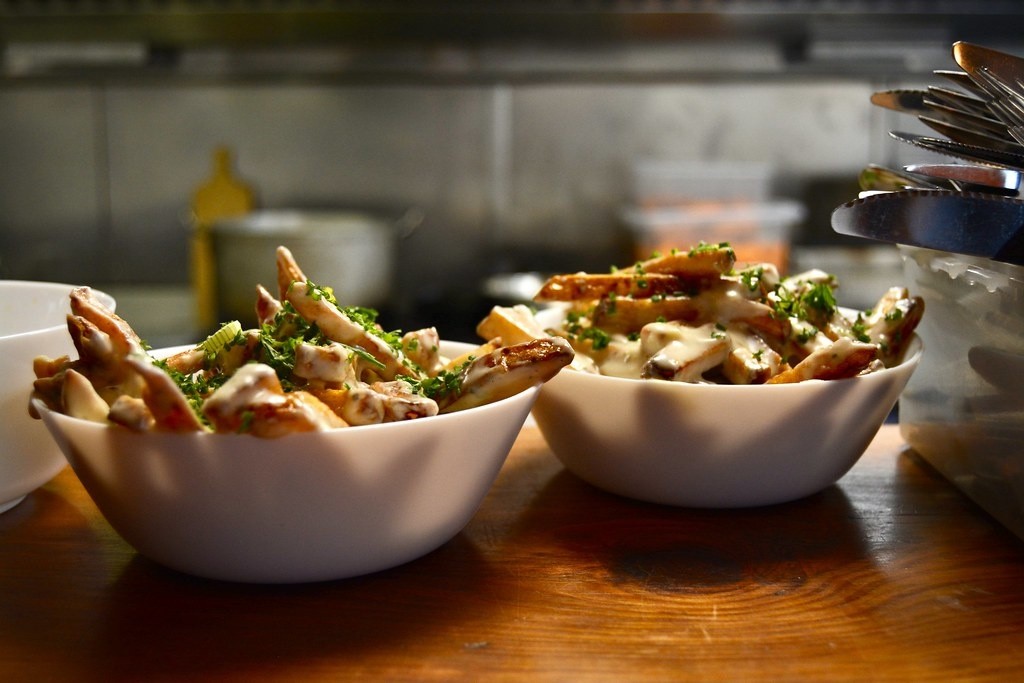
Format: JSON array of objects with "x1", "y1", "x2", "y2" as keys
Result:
[{"x1": 918, "y1": 69, "x2": 1024, "y2": 148}]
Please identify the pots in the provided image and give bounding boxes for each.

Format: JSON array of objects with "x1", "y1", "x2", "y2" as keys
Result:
[{"x1": 176, "y1": 202, "x2": 428, "y2": 324}]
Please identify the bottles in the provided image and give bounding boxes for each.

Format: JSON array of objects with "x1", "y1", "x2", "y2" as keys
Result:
[{"x1": 192, "y1": 146, "x2": 251, "y2": 331}]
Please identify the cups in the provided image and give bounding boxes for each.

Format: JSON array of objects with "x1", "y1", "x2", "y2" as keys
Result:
[{"x1": 620, "y1": 202, "x2": 803, "y2": 280}]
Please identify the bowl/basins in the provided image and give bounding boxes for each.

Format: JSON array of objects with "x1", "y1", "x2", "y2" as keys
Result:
[
  {"x1": 33, "y1": 341, "x2": 541, "y2": 583},
  {"x1": 530, "y1": 305, "x2": 923, "y2": 508},
  {"x1": 0, "y1": 279, "x2": 115, "y2": 511}
]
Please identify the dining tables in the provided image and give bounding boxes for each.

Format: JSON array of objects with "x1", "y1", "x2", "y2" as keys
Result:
[{"x1": 0, "y1": 420, "x2": 1024, "y2": 683}]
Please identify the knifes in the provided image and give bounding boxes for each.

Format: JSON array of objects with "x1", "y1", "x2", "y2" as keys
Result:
[
  {"x1": 951, "y1": 41, "x2": 1024, "y2": 86},
  {"x1": 831, "y1": 166, "x2": 1024, "y2": 267},
  {"x1": 872, "y1": 90, "x2": 1024, "y2": 172}
]
